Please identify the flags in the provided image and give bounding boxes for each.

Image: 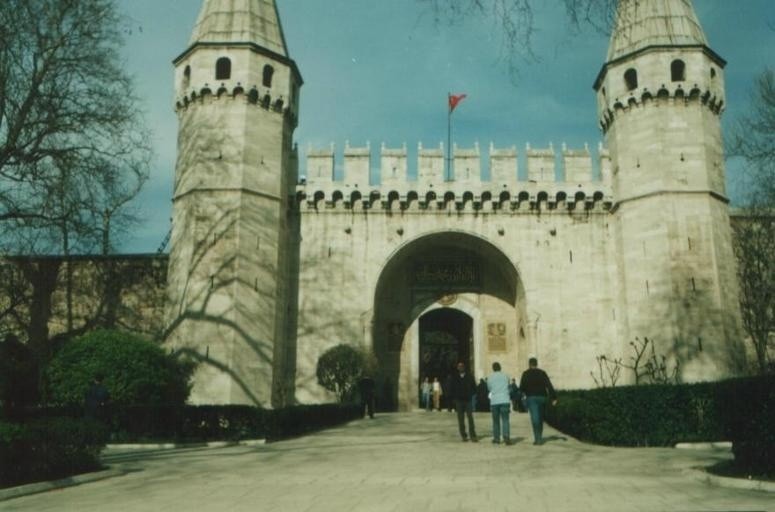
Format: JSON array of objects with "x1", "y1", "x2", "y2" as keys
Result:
[{"x1": 450, "y1": 93, "x2": 466, "y2": 112}]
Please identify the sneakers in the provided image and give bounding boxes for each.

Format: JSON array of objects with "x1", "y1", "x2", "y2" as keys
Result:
[
  {"x1": 492, "y1": 439, "x2": 511, "y2": 445},
  {"x1": 532, "y1": 441, "x2": 542, "y2": 445},
  {"x1": 461, "y1": 436, "x2": 478, "y2": 443}
]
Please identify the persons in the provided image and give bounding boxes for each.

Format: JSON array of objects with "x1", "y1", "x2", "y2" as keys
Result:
[
  {"x1": 486, "y1": 361, "x2": 511, "y2": 445},
  {"x1": 519, "y1": 357, "x2": 558, "y2": 446},
  {"x1": 446, "y1": 361, "x2": 479, "y2": 443},
  {"x1": 357, "y1": 370, "x2": 377, "y2": 419},
  {"x1": 420, "y1": 375, "x2": 519, "y2": 414}
]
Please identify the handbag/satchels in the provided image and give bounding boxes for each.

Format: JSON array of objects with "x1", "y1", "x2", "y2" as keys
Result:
[{"x1": 512, "y1": 388, "x2": 529, "y2": 413}]
